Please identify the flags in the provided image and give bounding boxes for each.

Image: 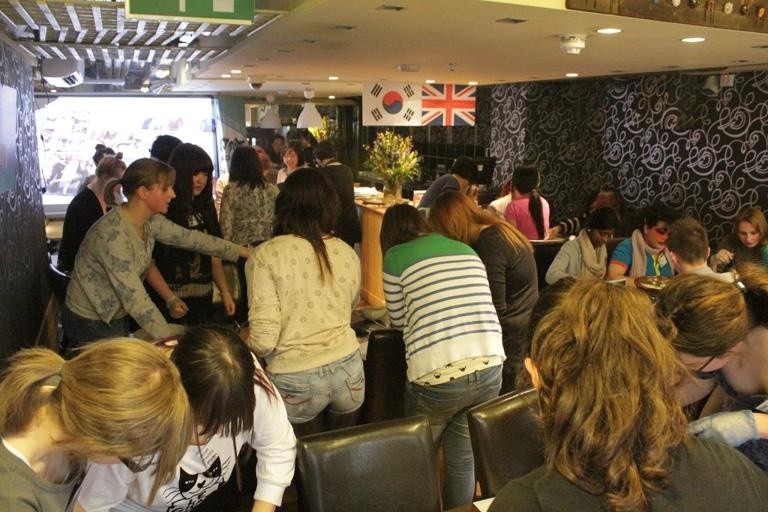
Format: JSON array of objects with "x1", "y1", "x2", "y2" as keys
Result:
[
  {"x1": 362, "y1": 83, "x2": 422, "y2": 126},
  {"x1": 422, "y1": 84, "x2": 475, "y2": 126}
]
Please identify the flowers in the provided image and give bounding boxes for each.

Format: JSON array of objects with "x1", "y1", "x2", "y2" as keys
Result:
[
  {"x1": 310, "y1": 116, "x2": 340, "y2": 156},
  {"x1": 362, "y1": 130, "x2": 424, "y2": 206}
]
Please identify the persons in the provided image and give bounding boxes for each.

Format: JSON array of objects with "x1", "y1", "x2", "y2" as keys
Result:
[
  {"x1": 504, "y1": 162, "x2": 546, "y2": 240},
  {"x1": 238, "y1": 167, "x2": 366, "y2": 434},
  {"x1": 488, "y1": 283, "x2": 767, "y2": 511},
  {"x1": 220, "y1": 146, "x2": 275, "y2": 245},
  {"x1": 276, "y1": 141, "x2": 305, "y2": 183},
  {"x1": 145, "y1": 144, "x2": 236, "y2": 320},
  {"x1": 657, "y1": 259, "x2": 768, "y2": 409},
  {"x1": 381, "y1": 203, "x2": 507, "y2": 511},
  {"x1": 608, "y1": 203, "x2": 674, "y2": 284},
  {"x1": 58, "y1": 154, "x2": 127, "y2": 297},
  {"x1": 313, "y1": 141, "x2": 361, "y2": 246},
  {"x1": 546, "y1": 208, "x2": 618, "y2": 286},
  {"x1": 77, "y1": 145, "x2": 116, "y2": 191},
  {"x1": 710, "y1": 207, "x2": 768, "y2": 271},
  {"x1": 73, "y1": 326, "x2": 296, "y2": 512},
  {"x1": 419, "y1": 157, "x2": 477, "y2": 210},
  {"x1": 150, "y1": 134, "x2": 178, "y2": 160},
  {"x1": 271, "y1": 134, "x2": 286, "y2": 160},
  {"x1": 66, "y1": 159, "x2": 253, "y2": 343},
  {"x1": 417, "y1": 190, "x2": 539, "y2": 396},
  {"x1": 548, "y1": 188, "x2": 624, "y2": 239},
  {"x1": 667, "y1": 219, "x2": 744, "y2": 288},
  {"x1": 0, "y1": 336, "x2": 193, "y2": 511}
]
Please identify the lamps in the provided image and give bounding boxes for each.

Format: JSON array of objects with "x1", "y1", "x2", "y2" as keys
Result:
[
  {"x1": 259, "y1": 94, "x2": 282, "y2": 129},
  {"x1": 297, "y1": 89, "x2": 323, "y2": 128}
]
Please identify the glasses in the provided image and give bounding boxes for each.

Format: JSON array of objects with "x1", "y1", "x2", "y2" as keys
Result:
[{"x1": 655, "y1": 227, "x2": 667, "y2": 234}]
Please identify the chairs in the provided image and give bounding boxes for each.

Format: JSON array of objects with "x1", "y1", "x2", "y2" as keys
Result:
[
  {"x1": 352, "y1": 330, "x2": 419, "y2": 424},
  {"x1": 523, "y1": 238, "x2": 570, "y2": 289},
  {"x1": 290, "y1": 415, "x2": 443, "y2": 512},
  {"x1": 467, "y1": 386, "x2": 548, "y2": 500}
]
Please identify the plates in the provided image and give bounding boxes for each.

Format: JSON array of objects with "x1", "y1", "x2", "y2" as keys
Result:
[{"x1": 634, "y1": 275, "x2": 673, "y2": 292}]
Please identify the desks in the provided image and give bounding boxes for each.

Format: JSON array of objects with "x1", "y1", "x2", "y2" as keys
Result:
[{"x1": 348, "y1": 191, "x2": 421, "y2": 310}]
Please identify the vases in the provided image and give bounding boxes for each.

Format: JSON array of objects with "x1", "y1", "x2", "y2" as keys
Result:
[{"x1": 381, "y1": 184, "x2": 402, "y2": 208}]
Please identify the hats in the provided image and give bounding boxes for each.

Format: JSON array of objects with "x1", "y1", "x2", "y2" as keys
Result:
[{"x1": 448, "y1": 157, "x2": 478, "y2": 180}]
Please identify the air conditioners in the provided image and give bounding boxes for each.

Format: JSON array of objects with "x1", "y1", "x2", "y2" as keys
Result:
[{"x1": 42, "y1": 56, "x2": 84, "y2": 87}]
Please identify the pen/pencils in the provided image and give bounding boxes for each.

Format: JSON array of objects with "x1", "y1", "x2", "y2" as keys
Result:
[{"x1": 233, "y1": 319, "x2": 241, "y2": 331}]
